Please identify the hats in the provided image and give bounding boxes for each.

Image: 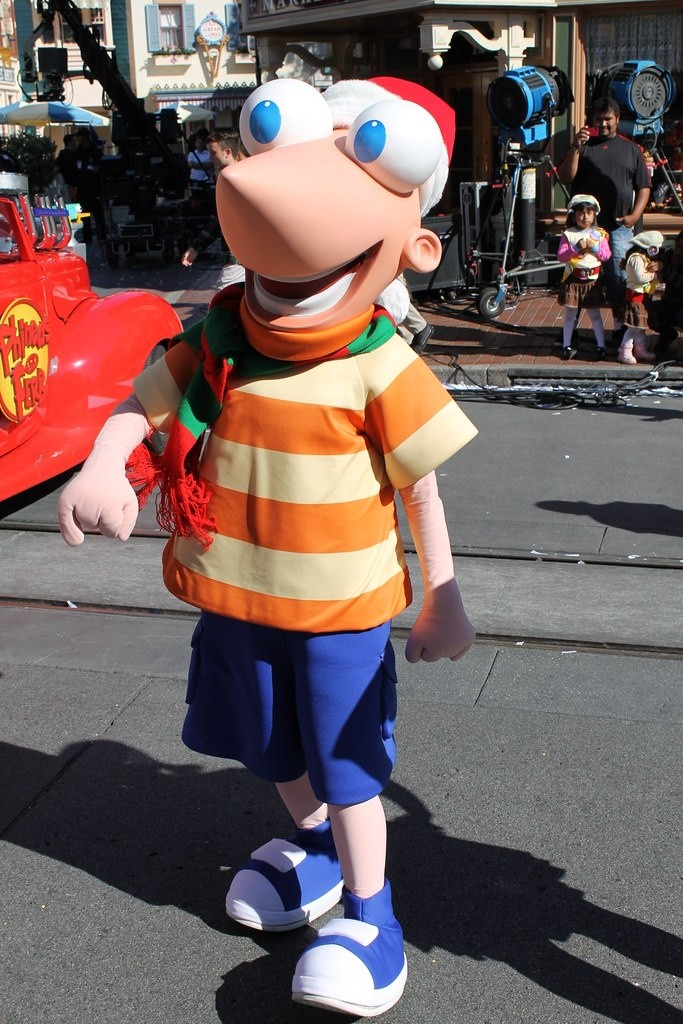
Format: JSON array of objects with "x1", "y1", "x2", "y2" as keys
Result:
[
  {"x1": 322, "y1": 77, "x2": 456, "y2": 219},
  {"x1": 73, "y1": 128, "x2": 90, "y2": 136},
  {"x1": 567, "y1": 194, "x2": 601, "y2": 216},
  {"x1": 628, "y1": 230, "x2": 664, "y2": 249}
]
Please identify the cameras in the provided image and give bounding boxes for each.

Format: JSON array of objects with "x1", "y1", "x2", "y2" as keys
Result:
[{"x1": 582, "y1": 127, "x2": 599, "y2": 137}]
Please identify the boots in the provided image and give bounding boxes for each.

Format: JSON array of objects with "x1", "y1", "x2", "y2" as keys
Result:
[
  {"x1": 618, "y1": 343, "x2": 637, "y2": 364},
  {"x1": 634, "y1": 342, "x2": 656, "y2": 360}
]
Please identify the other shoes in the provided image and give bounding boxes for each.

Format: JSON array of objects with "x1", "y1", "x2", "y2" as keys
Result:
[
  {"x1": 563, "y1": 345, "x2": 577, "y2": 360},
  {"x1": 79, "y1": 239, "x2": 92, "y2": 244},
  {"x1": 596, "y1": 346, "x2": 606, "y2": 359}
]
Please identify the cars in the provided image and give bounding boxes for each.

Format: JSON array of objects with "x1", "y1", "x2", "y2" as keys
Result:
[{"x1": 0, "y1": 171, "x2": 184, "y2": 506}]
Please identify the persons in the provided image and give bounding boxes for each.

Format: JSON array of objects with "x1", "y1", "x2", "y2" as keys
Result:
[
  {"x1": 59, "y1": 76, "x2": 478, "y2": 1017},
  {"x1": 561, "y1": 97, "x2": 652, "y2": 346},
  {"x1": 188, "y1": 137, "x2": 211, "y2": 186},
  {"x1": 182, "y1": 130, "x2": 251, "y2": 292},
  {"x1": 616, "y1": 231, "x2": 683, "y2": 364},
  {"x1": 60, "y1": 128, "x2": 104, "y2": 244},
  {"x1": 557, "y1": 194, "x2": 613, "y2": 362}
]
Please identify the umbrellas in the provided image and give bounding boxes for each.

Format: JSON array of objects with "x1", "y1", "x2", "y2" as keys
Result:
[
  {"x1": 151, "y1": 101, "x2": 218, "y2": 130},
  {"x1": 0, "y1": 100, "x2": 110, "y2": 135}
]
